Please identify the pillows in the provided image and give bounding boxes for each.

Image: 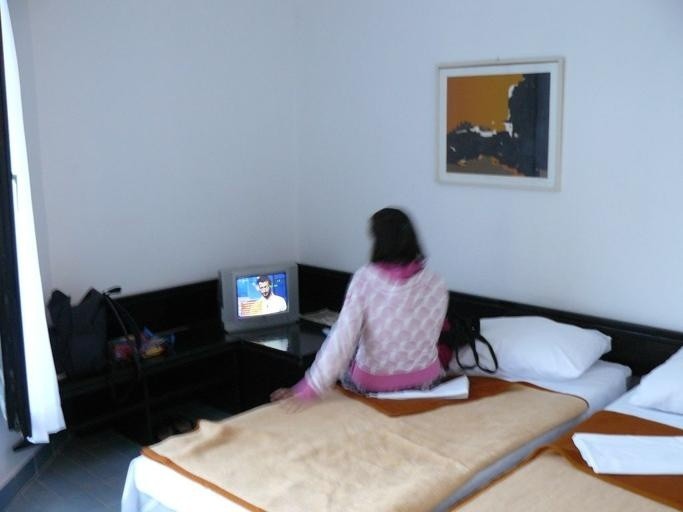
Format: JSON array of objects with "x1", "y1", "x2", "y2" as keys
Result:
[{"x1": 451, "y1": 313, "x2": 614, "y2": 376}]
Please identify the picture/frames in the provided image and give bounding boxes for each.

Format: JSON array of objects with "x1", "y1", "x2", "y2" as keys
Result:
[{"x1": 434, "y1": 53, "x2": 566, "y2": 193}]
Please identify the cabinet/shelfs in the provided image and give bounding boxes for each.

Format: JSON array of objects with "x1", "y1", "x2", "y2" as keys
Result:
[{"x1": 58, "y1": 318, "x2": 331, "y2": 446}]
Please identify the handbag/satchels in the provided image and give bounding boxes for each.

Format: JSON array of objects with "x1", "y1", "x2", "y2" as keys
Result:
[
  {"x1": 439, "y1": 302, "x2": 498, "y2": 374},
  {"x1": 48, "y1": 287, "x2": 142, "y2": 381}
]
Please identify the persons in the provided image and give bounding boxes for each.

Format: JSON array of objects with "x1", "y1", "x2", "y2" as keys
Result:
[
  {"x1": 248, "y1": 276, "x2": 286, "y2": 315},
  {"x1": 269, "y1": 205, "x2": 453, "y2": 416}
]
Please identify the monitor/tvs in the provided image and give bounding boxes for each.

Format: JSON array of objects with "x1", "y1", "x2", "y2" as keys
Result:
[{"x1": 219, "y1": 260, "x2": 299, "y2": 335}]
